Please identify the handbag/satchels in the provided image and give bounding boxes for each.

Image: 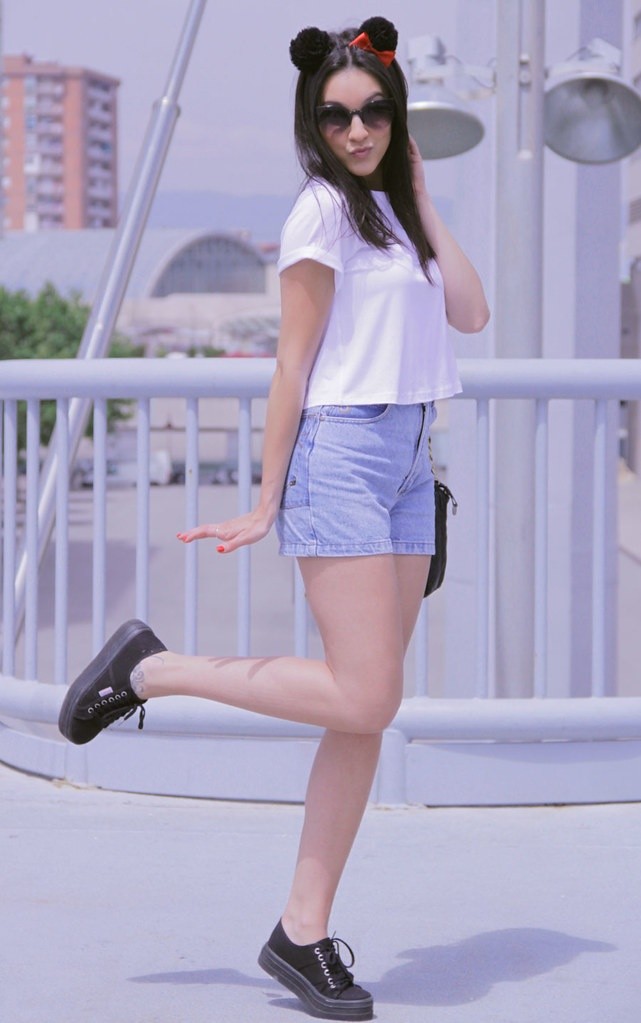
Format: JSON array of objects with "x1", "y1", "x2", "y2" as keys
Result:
[{"x1": 422, "y1": 480, "x2": 459, "y2": 598}]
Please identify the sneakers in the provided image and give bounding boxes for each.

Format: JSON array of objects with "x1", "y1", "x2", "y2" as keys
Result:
[
  {"x1": 257, "y1": 916, "x2": 374, "y2": 1021},
  {"x1": 58, "y1": 618, "x2": 167, "y2": 745}
]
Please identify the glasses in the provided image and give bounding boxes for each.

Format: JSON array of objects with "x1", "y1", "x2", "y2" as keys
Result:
[{"x1": 316, "y1": 97, "x2": 395, "y2": 134}]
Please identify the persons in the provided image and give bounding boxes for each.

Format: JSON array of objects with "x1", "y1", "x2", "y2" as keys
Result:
[{"x1": 54, "y1": 16, "x2": 490, "y2": 1023}]
